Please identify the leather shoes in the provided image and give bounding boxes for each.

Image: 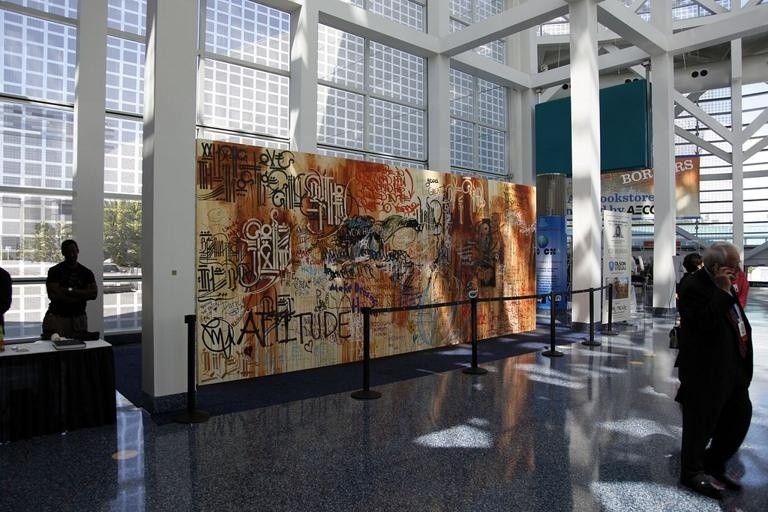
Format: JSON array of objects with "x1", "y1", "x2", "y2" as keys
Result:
[{"x1": 681, "y1": 470, "x2": 743, "y2": 496}]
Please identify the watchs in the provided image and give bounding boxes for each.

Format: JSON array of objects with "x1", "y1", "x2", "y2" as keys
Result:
[{"x1": 67, "y1": 287, "x2": 73, "y2": 296}]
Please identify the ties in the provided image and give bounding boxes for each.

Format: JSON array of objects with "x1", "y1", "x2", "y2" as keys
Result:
[{"x1": 728, "y1": 307, "x2": 747, "y2": 359}]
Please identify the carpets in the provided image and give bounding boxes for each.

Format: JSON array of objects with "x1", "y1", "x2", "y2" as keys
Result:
[{"x1": 114, "y1": 325, "x2": 604, "y2": 426}]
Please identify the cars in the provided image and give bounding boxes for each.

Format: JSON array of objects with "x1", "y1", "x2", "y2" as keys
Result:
[{"x1": 102, "y1": 263, "x2": 135, "y2": 293}]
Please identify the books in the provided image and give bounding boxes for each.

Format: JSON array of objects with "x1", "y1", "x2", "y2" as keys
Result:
[{"x1": 52, "y1": 340, "x2": 86, "y2": 350}]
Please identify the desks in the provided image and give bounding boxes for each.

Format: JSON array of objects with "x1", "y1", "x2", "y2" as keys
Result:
[{"x1": 0, "y1": 338, "x2": 116, "y2": 443}]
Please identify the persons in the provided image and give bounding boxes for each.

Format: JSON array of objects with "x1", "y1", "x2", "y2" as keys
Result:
[
  {"x1": 41, "y1": 239, "x2": 98, "y2": 339},
  {"x1": 674, "y1": 242, "x2": 754, "y2": 500},
  {"x1": 0, "y1": 267, "x2": 12, "y2": 344}
]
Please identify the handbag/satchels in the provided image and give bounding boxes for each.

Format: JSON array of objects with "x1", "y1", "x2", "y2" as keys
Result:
[{"x1": 669, "y1": 329, "x2": 681, "y2": 348}]
